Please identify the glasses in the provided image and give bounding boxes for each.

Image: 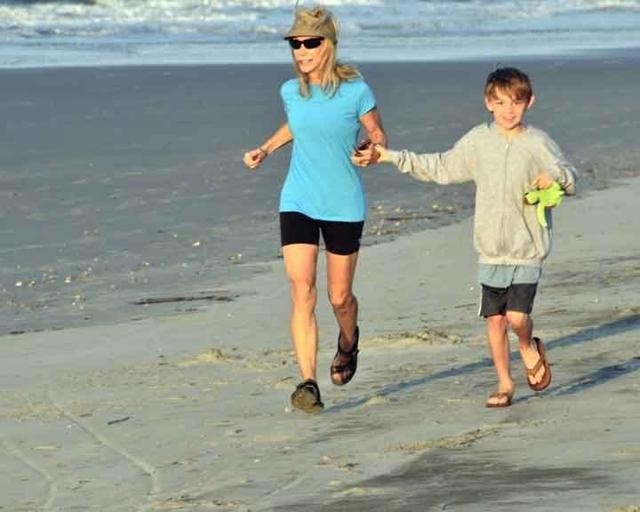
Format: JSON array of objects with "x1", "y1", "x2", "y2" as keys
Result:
[{"x1": 287, "y1": 36, "x2": 326, "y2": 51}]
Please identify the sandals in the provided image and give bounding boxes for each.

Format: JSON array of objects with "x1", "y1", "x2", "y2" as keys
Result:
[
  {"x1": 290, "y1": 378, "x2": 327, "y2": 416},
  {"x1": 329, "y1": 324, "x2": 361, "y2": 387}
]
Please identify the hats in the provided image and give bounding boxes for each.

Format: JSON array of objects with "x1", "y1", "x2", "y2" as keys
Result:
[{"x1": 284, "y1": 7, "x2": 341, "y2": 43}]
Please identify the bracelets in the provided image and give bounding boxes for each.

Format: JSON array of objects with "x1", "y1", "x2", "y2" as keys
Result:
[{"x1": 255, "y1": 145, "x2": 268, "y2": 161}]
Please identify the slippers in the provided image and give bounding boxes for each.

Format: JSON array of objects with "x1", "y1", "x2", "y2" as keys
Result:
[
  {"x1": 522, "y1": 334, "x2": 552, "y2": 391},
  {"x1": 484, "y1": 390, "x2": 515, "y2": 410}
]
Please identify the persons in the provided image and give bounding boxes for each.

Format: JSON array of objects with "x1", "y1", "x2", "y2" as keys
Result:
[
  {"x1": 363, "y1": 66, "x2": 578, "y2": 408},
  {"x1": 241, "y1": 5, "x2": 389, "y2": 417}
]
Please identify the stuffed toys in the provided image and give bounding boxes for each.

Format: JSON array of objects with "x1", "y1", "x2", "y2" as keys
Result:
[{"x1": 522, "y1": 180, "x2": 565, "y2": 228}]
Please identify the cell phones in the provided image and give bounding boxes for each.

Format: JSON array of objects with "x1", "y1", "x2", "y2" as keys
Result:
[{"x1": 358, "y1": 139, "x2": 372, "y2": 150}]
